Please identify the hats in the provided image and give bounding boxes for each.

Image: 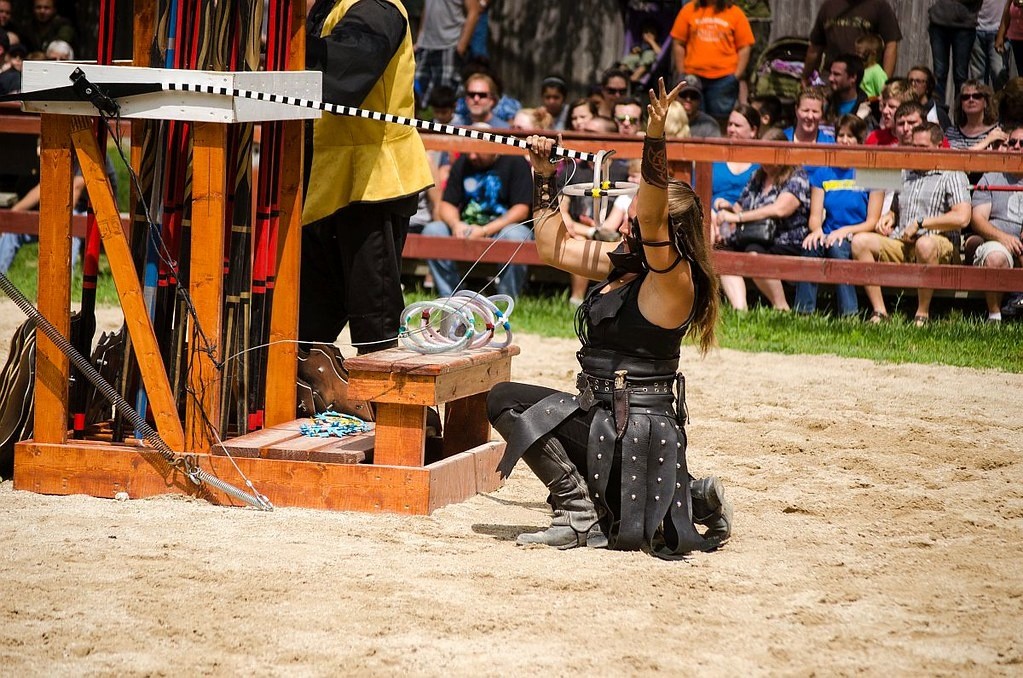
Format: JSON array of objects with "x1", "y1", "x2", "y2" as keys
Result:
[{"x1": 678, "y1": 74, "x2": 704, "y2": 101}]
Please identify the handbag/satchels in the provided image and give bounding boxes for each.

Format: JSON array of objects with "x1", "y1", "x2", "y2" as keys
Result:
[{"x1": 728, "y1": 218, "x2": 777, "y2": 250}]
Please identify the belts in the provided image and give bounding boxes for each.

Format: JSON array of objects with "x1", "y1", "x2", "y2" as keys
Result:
[{"x1": 575, "y1": 372, "x2": 674, "y2": 411}]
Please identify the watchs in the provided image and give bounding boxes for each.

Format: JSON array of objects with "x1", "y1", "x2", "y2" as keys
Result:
[{"x1": 916, "y1": 217, "x2": 924, "y2": 228}]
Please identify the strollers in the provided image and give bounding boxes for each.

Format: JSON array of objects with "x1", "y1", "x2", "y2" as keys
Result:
[{"x1": 748, "y1": 36, "x2": 825, "y2": 132}]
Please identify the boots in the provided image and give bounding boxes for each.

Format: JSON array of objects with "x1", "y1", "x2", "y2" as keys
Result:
[
  {"x1": 688, "y1": 478, "x2": 733, "y2": 545},
  {"x1": 492, "y1": 411, "x2": 609, "y2": 549}
]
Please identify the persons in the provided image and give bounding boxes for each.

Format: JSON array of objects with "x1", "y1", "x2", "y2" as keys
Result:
[
  {"x1": 0, "y1": 0, "x2": 77, "y2": 208},
  {"x1": 994, "y1": 0, "x2": 1023, "y2": 77},
  {"x1": 801, "y1": 0, "x2": 903, "y2": 83},
  {"x1": 625, "y1": 0, "x2": 681, "y2": 93},
  {"x1": 485, "y1": 77, "x2": 733, "y2": 546},
  {"x1": 927, "y1": 0, "x2": 983, "y2": 103},
  {"x1": 854, "y1": 33, "x2": 889, "y2": 100},
  {"x1": 260, "y1": 0, "x2": 434, "y2": 355},
  {"x1": 404, "y1": 54, "x2": 1023, "y2": 326},
  {"x1": 967, "y1": 0, "x2": 1012, "y2": 93},
  {"x1": 0, "y1": 146, "x2": 117, "y2": 281},
  {"x1": 669, "y1": 0, "x2": 756, "y2": 119},
  {"x1": 403, "y1": 0, "x2": 491, "y2": 111}
]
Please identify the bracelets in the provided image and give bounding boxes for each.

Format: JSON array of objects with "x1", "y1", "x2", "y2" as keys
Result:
[{"x1": 739, "y1": 212, "x2": 743, "y2": 217}]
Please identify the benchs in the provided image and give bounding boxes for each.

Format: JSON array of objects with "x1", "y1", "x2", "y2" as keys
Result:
[{"x1": 341, "y1": 343, "x2": 520, "y2": 469}]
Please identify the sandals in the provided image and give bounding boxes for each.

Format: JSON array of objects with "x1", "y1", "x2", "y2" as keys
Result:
[
  {"x1": 870, "y1": 313, "x2": 890, "y2": 326},
  {"x1": 914, "y1": 316, "x2": 929, "y2": 328}
]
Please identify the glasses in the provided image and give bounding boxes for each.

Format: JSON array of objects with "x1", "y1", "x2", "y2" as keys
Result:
[
  {"x1": 466, "y1": 91, "x2": 493, "y2": 98},
  {"x1": 909, "y1": 79, "x2": 928, "y2": 86},
  {"x1": 614, "y1": 115, "x2": 641, "y2": 125},
  {"x1": 960, "y1": 93, "x2": 985, "y2": 101},
  {"x1": 679, "y1": 91, "x2": 701, "y2": 100},
  {"x1": 1008, "y1": 139, "x2": 1023, "y2": 148},
  {"x1": 604, "y1": 87, "x2": 628, "y2": 97}
]
